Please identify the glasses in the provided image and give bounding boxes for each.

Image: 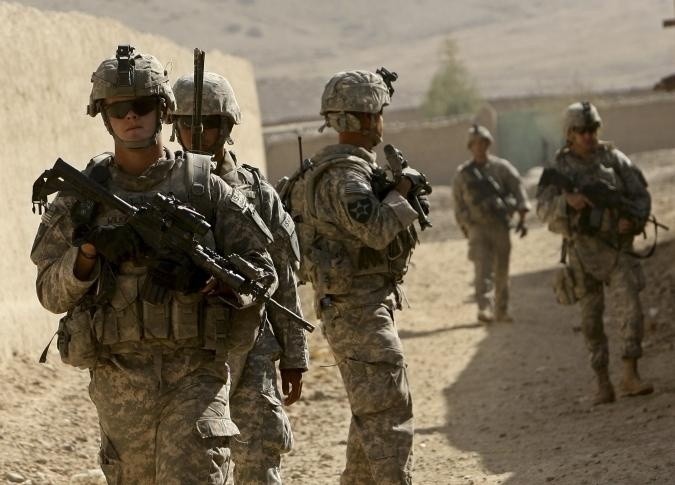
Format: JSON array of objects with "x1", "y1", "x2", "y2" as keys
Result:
[
  {"x1": 101, "y1": 97, "x2": 157, "y2": 119},
  {"x1": 182, "y1": 116, "x2": 217, "y2": 127}
]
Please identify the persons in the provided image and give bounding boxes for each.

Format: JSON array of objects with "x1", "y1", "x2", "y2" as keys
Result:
[
  {"x1": 31, "y1": 42, "x2": 278, "y2": 484},
  {"x1": 451, "y1": 125, "x2": 530, "y2": 323},
  {"x1": 165, "y1": 73, "x2": 309, "y2": 485},
  {"x1": 535, "y1": 102, "x2": 669, "y2": 407},
  {"x1": 276, "y1": 66, "x2": 433, "y2": 485}
]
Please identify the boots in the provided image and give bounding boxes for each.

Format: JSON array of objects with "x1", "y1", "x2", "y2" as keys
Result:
[
  {"x1": 620, "y1": 360, "x2": 652, "y2": 396},
  {"x1": 592, "y1": 369, "x2": 614, "y2": 404}
]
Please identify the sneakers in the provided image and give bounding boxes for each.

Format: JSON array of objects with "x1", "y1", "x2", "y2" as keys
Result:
[{"x1": 477, "y1": 309, "x2": 514, "y2": 323}]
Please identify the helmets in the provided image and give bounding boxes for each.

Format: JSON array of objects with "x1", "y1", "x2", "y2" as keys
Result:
[
  {"x1": 465, "y1": 126, "x2": 495, "y2": 149},
  {"x1": 88, "y1": 46, "x2": 177, "y2": 116},
  {"x1": 320, "y1": 68, "x2": 398, "y2": 113},
  {"x1": 165, "y1": 72, "x2": 242, "y2": 125},
  {"x1": 564, "y1": 102, "x2": 600, "y2": 131}
]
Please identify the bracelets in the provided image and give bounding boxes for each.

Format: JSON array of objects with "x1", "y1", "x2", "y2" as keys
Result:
[{"x1": 77, "y1": 248, "x2": 100, "y2": 264}]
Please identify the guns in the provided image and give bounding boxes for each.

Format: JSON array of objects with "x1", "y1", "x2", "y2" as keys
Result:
[
  {"x1": 50, "y1": 158, "x2": 314, "y2": 334},
  {"x1": 460, "y1": 159, "x2": 512, "y2": 218},
  {"x1": 190, "y1": 49, "x2": 206, "y2": 152},
  {"x1": 384, "y1": 143, "x2": 432, "y2": 230},
  {"x1": 538, "y1": 168, "x2": 669, "y2": 232}
]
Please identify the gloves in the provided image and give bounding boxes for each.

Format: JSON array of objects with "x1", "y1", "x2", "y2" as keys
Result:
[
  {"x1": 154, "y1": 260, "x2": 214, "y2": 293},
  {"x1": 91, "y1": 225, "x2": 145, "y2": 265}
]
